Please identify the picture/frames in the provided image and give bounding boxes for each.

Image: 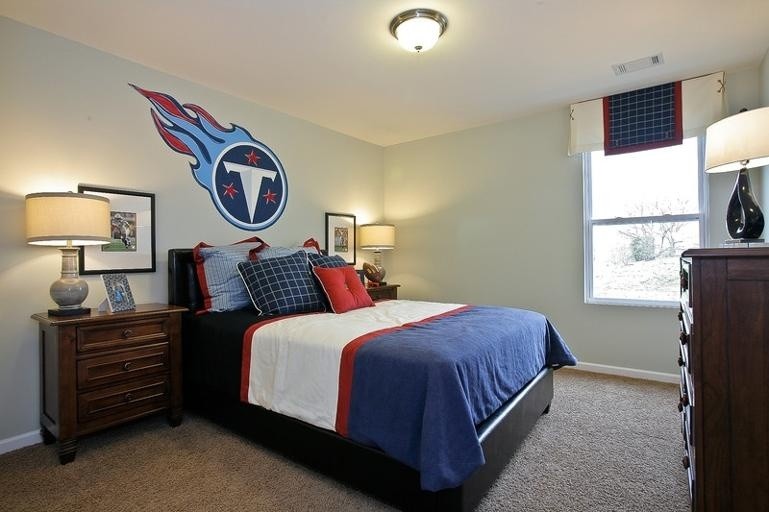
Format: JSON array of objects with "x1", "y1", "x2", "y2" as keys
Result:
[
  {"x1": 97, "y1": 271, "x2": 137, "y2": 313},
  {"x1": 354, "y1": 268, "x2": 366, "y2": 287},
  {"x1": 74, "y1": 184, "x2": 158, "y2": 276},
  {"x1": 323, "y1": 211, "x2": 359, "y2": 268}
]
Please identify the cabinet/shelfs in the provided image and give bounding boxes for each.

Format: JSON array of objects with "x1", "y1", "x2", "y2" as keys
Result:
[{"x1": 670, "y1": 242, "x2": 768, "y2": 510}]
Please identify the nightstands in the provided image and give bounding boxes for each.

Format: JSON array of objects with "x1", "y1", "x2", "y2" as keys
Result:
[
  {"x1": 31, "y1": 300, "x2": 193, "y2": 466},
  {"x1": 363, "y1": 283, "x2": 402, "y2": 300}
]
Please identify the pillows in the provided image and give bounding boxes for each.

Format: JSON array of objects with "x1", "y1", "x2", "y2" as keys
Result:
[
  {"x1": 190, "y1": 235, "x2": 272, "y2": 315},
  {"x1": 247, "y1": 240, "x2": 322, "y2": 263},
  {"x1": 311, "y1": 262, "x2": 378, "y2": 315},
  {"x1": 234, "y1": 250, "x2": 329, "y2": 319},
  {"x1": 306, "y1": 252, "x2": 349, "y2": 272}
]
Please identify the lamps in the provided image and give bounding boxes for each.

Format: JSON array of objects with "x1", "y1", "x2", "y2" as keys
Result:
[
  {"x1": 388, "y1": 5, "x2": 450, "y2": 56},
  {"x1": 358, "y1": 219, "x2": 398, "y2": 288},
  {"x1": 22, "y1": 188, "x2": 113, "y2": 316},
  {"x1": 701, "y1": 104, "x2": 768, "y2": 244}
]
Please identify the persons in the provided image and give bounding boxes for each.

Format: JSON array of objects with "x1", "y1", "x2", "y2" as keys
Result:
[{"x1": 111, "y1": 214, "x2": 131, "y2": 248}]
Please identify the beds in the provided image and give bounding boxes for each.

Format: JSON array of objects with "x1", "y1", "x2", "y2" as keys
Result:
[{"x1": 164, "y1": 246, "x2": 578, "y2": 512}]
